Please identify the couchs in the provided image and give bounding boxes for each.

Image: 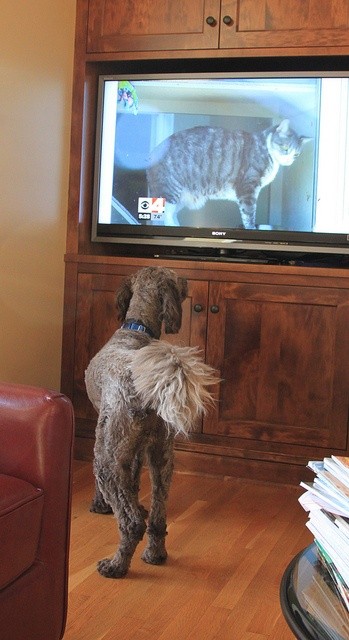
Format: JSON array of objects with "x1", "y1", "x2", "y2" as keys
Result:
[{"x1": 1, "y1": 385, "x2": 76, "y2": 639}]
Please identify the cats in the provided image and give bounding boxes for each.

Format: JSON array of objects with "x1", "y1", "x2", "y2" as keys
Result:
[{"x1": 145, "y1": 119, "x2": 314, "y2": 231}]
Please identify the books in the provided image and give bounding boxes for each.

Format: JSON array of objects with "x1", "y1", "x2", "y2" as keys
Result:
[{"x1": 298, "y1": 454, "x2": 348, "y2": 640}]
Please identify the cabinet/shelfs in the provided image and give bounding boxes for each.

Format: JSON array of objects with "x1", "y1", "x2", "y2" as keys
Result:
[
  {"x1": 62, "y1": 254, "x2": 349, "y2": 485},
  {"x1": 76, "y1": 0, "x2": 348, "y2": 61}
]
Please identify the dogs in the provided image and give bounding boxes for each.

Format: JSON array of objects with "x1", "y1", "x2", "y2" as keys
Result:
[{"x1": 85, "y1": 264, "x2": 226, "y2": 578}]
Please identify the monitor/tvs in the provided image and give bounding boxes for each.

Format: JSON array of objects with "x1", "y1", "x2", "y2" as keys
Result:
[{"x1": 91, "y1": 71, "x2": 349, "y2": 265}]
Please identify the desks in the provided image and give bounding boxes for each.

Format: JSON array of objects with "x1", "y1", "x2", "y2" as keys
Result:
[{"x1": 279, "y1": 542, "x2": 349, "y2": 640}]
